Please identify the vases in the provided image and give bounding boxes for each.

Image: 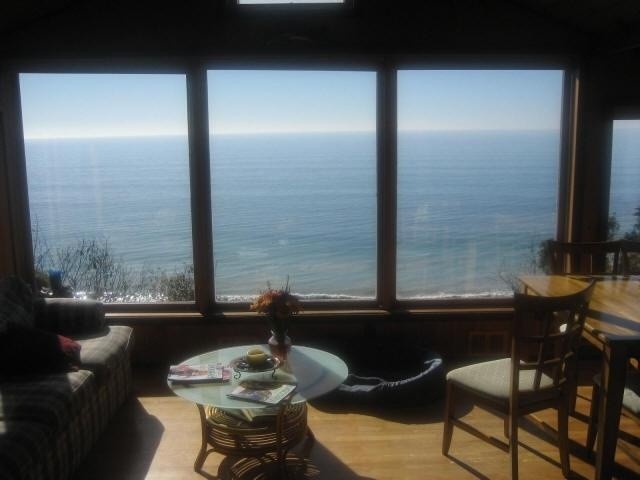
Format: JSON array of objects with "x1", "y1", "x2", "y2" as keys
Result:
[{"x1": 268, "y1": 329, "x2": 292, "y2": 357}]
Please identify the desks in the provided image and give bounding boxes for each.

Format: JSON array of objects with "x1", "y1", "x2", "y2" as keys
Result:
[{"x1": 514, "y1": 274, "x2": 640, "y2": 479}]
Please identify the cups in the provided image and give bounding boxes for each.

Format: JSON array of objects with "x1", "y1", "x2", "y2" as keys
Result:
[{"x1": 245, "y1": 347, "x2": 269, "y2": 366}]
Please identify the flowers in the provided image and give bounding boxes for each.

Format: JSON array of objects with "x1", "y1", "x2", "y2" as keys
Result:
[{"x1": 249, "y1": 276, "x2": 305, "y2": 358}]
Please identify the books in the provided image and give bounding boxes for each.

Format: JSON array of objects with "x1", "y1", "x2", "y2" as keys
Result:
[
  {"x1": 283, "y1": 390, "x2": 296, "y2": 401},
  {"x1": 226, "y1": 381, "x2": 297, "y2": 407},
  {"x1": 168, "y1": 364, "x2": 224, "y2": 383},
  {"x1": 221, "y1": 364, "x2": 233, "y2": 382}
]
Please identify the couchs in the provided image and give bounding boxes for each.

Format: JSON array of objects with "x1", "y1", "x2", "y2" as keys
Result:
[{"x1": 1, "y1": 273, "x2": 134, "y2": 480}]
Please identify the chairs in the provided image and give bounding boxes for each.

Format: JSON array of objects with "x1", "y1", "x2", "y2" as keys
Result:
[
  {"x1": 531, "y1": 239, "x2": 621, "y2": 274},
  {"x1": 438, "y1": 280, "x2": 597, "y2": 480}
]
